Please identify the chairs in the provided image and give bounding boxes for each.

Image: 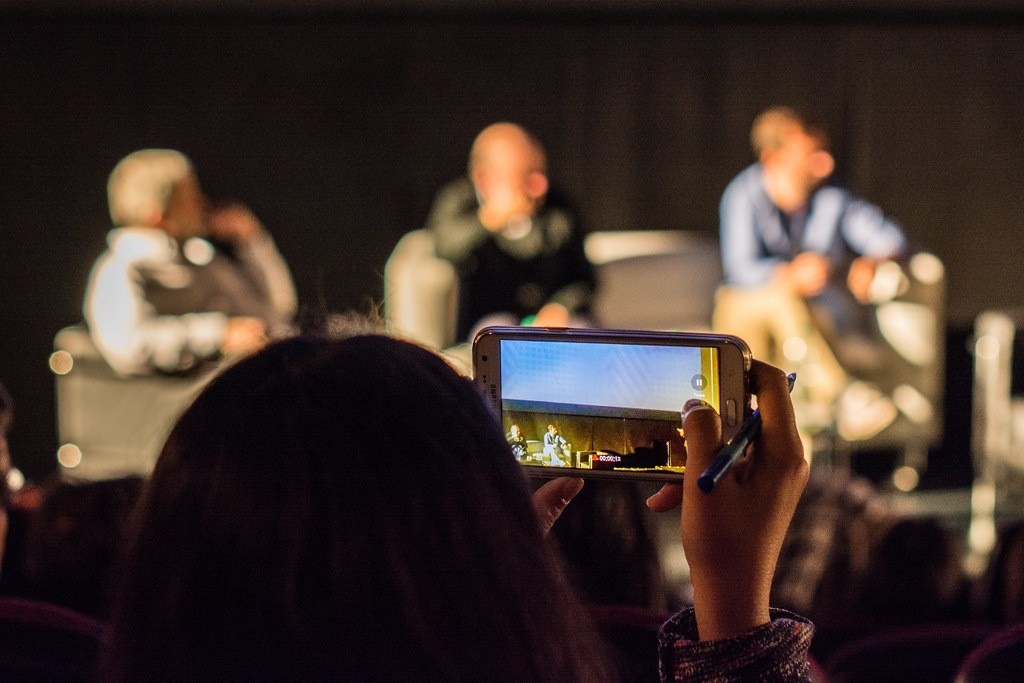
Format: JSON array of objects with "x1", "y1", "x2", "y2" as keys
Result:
[
  {"x1": 56, "y1": 321, "x2": 224, "y2": 483},
  {"x1": 966, "y1": 310, "x2": 1024, "y2": 573}
]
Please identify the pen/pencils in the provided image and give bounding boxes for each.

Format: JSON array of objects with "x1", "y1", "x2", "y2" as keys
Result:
[{"x1": 697, "y1": 372, "x2": 798, "y2": 495}]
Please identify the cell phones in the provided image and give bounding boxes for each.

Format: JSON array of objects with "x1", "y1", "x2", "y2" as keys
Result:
[{"x1": 473, "y1": 325, "x2": 752, "y2": 484}]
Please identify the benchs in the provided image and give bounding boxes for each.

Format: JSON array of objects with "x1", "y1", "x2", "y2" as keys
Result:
[{"x1": 380, "y1": 230, "x2": 948, "y2": 485}]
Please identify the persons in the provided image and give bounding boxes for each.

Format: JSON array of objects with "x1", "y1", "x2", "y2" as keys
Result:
[
  {"x1": 544, "y1": 424, "x2": 568, "y2": 467},
  {"x1": 505, "y1": 425, "x2": 528, "y2": 464},
  {"x1": 719, "y1": 105, "x2": 906, "y2": 441},
  {"x1": 1, "y1": 334, "x2": 1023, "y2": 683},
  {"x1": 81, "y1": 149, "x2": 297, "y2": 376},
  {"x1": 440, "y1": 121, "x2": 595, "y2": 344}
]
